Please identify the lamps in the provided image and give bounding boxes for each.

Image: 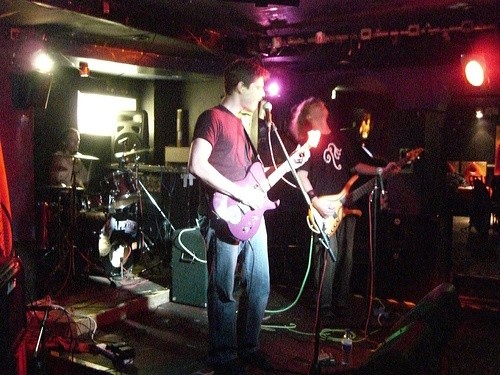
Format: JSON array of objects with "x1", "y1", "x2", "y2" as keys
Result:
[{"x1": 79, "y1": 63, "x2": 90, "y2": 78}]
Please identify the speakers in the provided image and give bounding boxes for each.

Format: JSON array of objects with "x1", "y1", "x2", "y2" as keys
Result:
[
  {"x1": 169, "y1": 228, "x2": 210, "y2": 307},
  {"x1": 0, "y1": 256, "x2": 28, "y2": 371},
  {"x1": 358, "y1": 282, "x2": 463, "y2": 375}
]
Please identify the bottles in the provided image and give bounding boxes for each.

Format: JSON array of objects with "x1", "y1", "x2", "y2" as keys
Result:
[{"x1": 341, "y1": 329, "x2": 353, "y2": 364}]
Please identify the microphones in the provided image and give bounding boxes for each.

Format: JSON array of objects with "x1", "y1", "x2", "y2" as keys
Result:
[
  {"x1": 23, "y1": 304, "x2": 61, "y2": 311},
  {"x1": 117, "y1": 136, "x2": 129, "y2": 145},
  {"x1": 263, "y1": 102, "x2": 273, "y2": 131},
  {"x1": 376, "y1": 167, "x2": 385, "y2": 195}
]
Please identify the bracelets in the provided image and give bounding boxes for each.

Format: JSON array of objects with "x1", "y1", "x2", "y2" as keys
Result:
[{"x1": 376, "y1": 167, "x2": 383, "y2": 175}]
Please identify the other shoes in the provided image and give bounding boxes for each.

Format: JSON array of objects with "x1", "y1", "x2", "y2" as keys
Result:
[
  {"x1": 238, "y1": 348, "x2": 270, "y2": 370},
  {"x1": 210, "y1": 359, "x2": 243, "y2": 375},
  {"x1": 319, "y1": 306, "x2": 334, "y2": 321},
  {"x1": 337, "y1": 302, "x2": 354, "y2": 311}
]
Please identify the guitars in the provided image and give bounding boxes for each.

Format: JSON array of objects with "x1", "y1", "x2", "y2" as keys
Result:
[
  {"x1": 209, "y1": 130, "x2": 321, "y2": 242},
  {"x1": 307, "y1": 149, "x2": 423, "y2": 237}
]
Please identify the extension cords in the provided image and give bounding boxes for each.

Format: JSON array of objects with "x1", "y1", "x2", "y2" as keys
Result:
[{"x1": 97, "y1": 343, "x2": 134, "y2": 365}]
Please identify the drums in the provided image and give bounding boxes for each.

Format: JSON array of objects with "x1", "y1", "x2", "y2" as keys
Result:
[
  {"x1": 74, "y1": 212, "x2": 114, "y2": 255},
  {"x1": 102, "y1": 230, "x2": 138, "y2": 272}
]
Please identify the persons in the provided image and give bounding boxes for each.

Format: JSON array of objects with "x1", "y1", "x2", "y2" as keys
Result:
[
  {"x1": 188, "y1": 58, "x2": 312, "y2": 375},
  {"x1": 289, "y1": 97, "x2": 400, "y2": 334},
  {"x1": 48, "y1": 128, "x2": 87, "y2": 186}
]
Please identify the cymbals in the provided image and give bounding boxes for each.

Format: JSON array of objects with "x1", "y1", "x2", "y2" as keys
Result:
[
  {"x1": 43, "y1": 184, "x2": 84, "y2": 192},
  {"x1": 102, "y1": 196, "x2": 139, "y2": 211},
  {"x1": 56, "y1": 151, "x2": 99, "y2": 163}
]
